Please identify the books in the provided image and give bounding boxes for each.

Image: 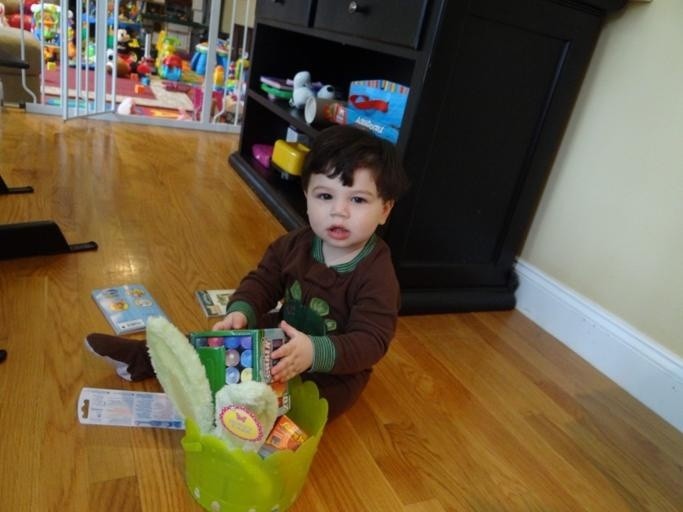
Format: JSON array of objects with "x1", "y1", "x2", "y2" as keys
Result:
[
  {"x1": 194, "y1": 289, "x2": 243, "y2": 318},
  {"x1": 89, "y1": 281, "x2": 169, "y2": 337}
]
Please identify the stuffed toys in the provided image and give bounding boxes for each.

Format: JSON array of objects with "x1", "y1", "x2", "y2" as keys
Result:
[{"x1": 258, "y1": 71, "x2": 337, "y2": 108}]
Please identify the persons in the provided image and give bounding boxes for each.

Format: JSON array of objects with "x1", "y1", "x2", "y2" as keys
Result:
[{"x1": 84, "y1": 123, "x2": 402, "y2": 424}]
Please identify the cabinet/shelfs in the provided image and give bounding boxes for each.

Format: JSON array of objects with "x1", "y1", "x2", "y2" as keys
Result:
[{"x1": 228, "y1": 1, "x2": 648, "y2": 319}]
